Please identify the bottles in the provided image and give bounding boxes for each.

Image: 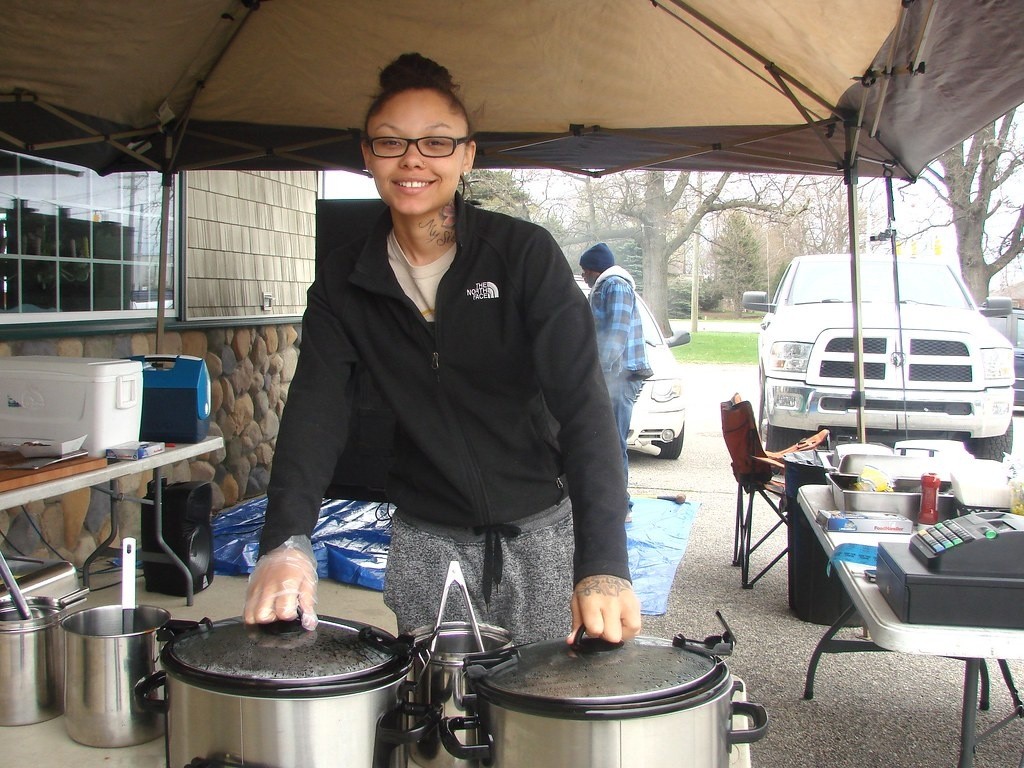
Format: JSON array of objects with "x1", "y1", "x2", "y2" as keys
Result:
[{"x1": 918, "y1": 449, "x2": 940, "y2": 525}]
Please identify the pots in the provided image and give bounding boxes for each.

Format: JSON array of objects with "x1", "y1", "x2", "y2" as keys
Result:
[
  {"x1": 406, "y1": 616, "x2": 513, "y2": 768},
  {"x1": 1, "y1": 592, "x2": 66, "y2": 728},
  {"x1": 134, "y1": 608, "x2": 428, "y2": 767},
  {"x1": 439, "y1": 608, "x2": 766, "y2": 767},
  {"x1": 59, "y1": 537, "x2": 177, "y2": 748}
]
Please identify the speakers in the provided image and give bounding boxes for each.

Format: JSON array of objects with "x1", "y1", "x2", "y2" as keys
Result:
[{"x1": 140, "y1": 477, "x2": 214, "y2": 596}]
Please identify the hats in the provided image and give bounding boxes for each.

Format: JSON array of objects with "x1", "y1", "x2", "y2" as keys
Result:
[{"x1": 579, "y1": 242, "x2": 614, "y2": 272}]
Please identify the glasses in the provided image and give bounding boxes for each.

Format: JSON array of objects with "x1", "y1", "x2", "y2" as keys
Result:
[{"x1": 365, "y1": 133, "x2": 470, "y2": 158}]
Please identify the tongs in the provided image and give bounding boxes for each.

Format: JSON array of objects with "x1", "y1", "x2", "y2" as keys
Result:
[{"x1": 426, "y1": 559, "x2": 486, "y2": 653}]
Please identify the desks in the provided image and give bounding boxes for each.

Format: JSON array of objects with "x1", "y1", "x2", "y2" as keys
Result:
[
  {"x1": 792, "y1": 475, "x2": 1024, "y2": 767},
  {"x1": 0, "y1": 432, "x2": 226, "y2": 602}
]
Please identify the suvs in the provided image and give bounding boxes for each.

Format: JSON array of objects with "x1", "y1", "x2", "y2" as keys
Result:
[
  {"x1": 741, "y1": 251, "x2": 1016, "y2": 476},
  {"x1": 570, "y1": 274, "x2": 693, "y2": 460}
]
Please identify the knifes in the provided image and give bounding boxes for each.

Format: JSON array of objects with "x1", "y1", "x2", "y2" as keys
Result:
[{"x1": 7, "y1": 450, "x2": 87, "y2": 471}]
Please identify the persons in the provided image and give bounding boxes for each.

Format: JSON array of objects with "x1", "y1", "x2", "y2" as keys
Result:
[
  {"x1": 574, "y1": 234, "x2": 654, "y2": 515},
  {"x1": 236, "y1": 46, "x2": 646, "y2": 660}
]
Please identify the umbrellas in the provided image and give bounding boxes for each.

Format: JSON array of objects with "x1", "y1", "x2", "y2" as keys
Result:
[{"x1": 2, "y1": 0, "x2": 1022, "y2": 182}]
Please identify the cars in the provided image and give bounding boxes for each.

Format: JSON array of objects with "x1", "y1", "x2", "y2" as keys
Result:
[{"x1": 979, "y1": 306, "x2": 1024, "y2": 406}]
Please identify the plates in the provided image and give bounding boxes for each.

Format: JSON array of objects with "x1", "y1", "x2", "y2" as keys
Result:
[{"x1": 12, "y1": 434, "x2": 90, "y2": 457}]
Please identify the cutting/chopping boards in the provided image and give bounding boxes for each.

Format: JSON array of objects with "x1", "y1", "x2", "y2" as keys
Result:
[{"x1": 0, "y1": 455, "x2": 110, "y2": 493}]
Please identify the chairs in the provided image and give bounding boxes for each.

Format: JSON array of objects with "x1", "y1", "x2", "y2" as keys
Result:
[{"x1": 720, "y1": 392, "x2": 834, "y2": 586}]
